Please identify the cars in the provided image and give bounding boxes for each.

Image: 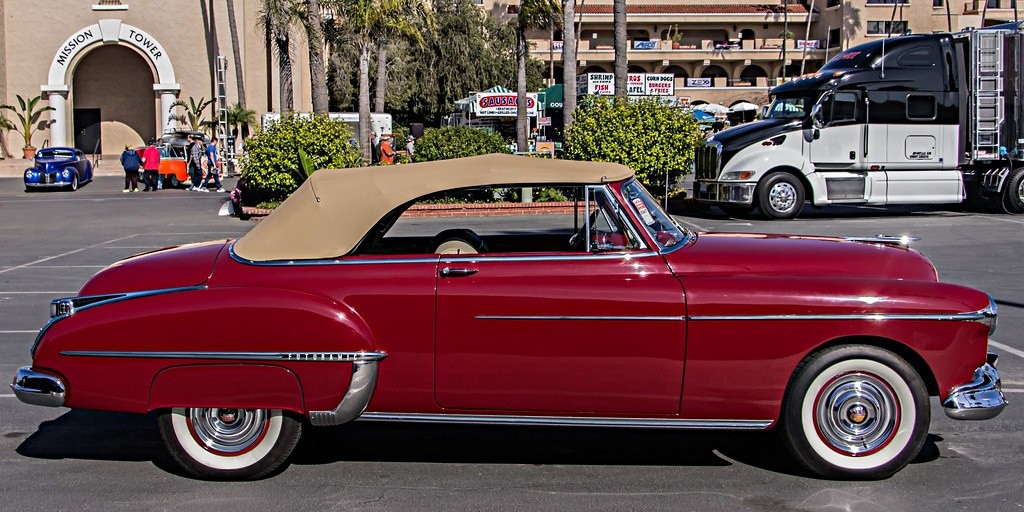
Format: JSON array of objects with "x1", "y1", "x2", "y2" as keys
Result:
[
  {"x1": 132, "y1": 132, "x2": 228, "y2": 188},
  {"x1": 23, "y1": 147, "x2": 93, "y2": 191},
  {"x1": 9, "y1": 152, "x2": 1011, "y2": 483}
]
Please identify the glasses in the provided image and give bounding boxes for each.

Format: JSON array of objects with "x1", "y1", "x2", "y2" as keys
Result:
[{"x1": 373, "y1": 134, "x2": 377, "y2": 137}]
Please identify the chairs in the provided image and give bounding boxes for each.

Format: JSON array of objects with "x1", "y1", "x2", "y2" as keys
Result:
[{"x1": 432, "y1": 229, "x2": 494, "y2": 254}]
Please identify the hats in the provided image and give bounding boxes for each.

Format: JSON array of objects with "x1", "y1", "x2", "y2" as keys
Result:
[
  {"x1": 370, "y1": 132, "x2": 376, "y2": 136},
  {"x1": 405, "y1": 134, "x2": 415, "y2": 141}
]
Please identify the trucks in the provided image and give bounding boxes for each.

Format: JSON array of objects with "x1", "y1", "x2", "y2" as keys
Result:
[{"x1": 692, "y1": 21, "x2": 1024, "y2": 216}]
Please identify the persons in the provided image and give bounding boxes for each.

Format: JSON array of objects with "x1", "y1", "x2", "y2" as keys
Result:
[
  {"x1": 186, "y1": 134, "x2": 227, "y2": 193},
  {"x1": 142, "y1": 140, "x2": 160, "y2": 191},
  {"x1": 370, "y1": 130, "x2": 396, "y2": 166},
  {"x1": 405, "y1": 134, "x2": 417, "y2": 162},
  {"x1": 119, "y1": 143, "x2": 144, "y2": 193}
]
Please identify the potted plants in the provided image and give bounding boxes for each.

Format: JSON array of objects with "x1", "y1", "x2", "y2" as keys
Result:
[
  {"x1": 0, "y1": 94, "x2": 56, "y2": 159},
  {"x1": 666, "y1": 24, "x2": 683, "y2": 49}
]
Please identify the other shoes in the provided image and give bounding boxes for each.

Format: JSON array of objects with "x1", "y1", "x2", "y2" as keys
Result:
[
  {"x1": 151, "y1": 188, "x2": 156, "y2": 192},
  {"x1": 198, "y1": 188, "x2": 209, "y2": 192},
  {"x1": 123, "y1": 189, "x2": 130, "y2": 192},
  {"x1": 217, "y1": 187, "x2": 226, "y2": 192},
  {"x1": 191, "y1": 186, "x2": 200, "y2": 190},
  {"x1": 133, "y1": 188, "x2": 138, "y2": 193},
  {"x1": 186, "y1": 186, "x2": 192, "y2": 191},
  {"x1": 143, "y1": 188, "x2": 149, "y2": 192}
]
